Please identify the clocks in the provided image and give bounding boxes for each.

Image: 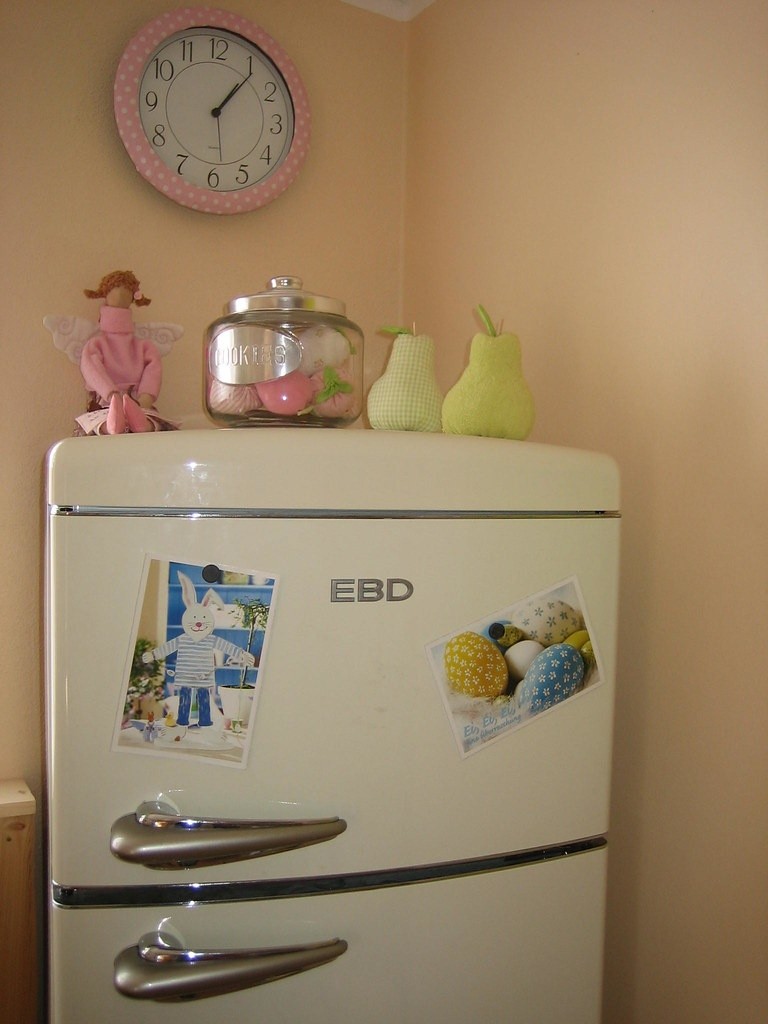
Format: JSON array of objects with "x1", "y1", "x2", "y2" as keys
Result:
[{"x1": 112, "y1": 4, "x2": 312, "y2": 217}]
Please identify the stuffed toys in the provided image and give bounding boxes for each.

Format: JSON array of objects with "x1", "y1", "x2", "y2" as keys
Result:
[
  {"x1": 441, "y1": 304, "x2": 535, "y2": 441},
  {"x1": 44, "y1": 271, "x2": 184, "y2": 437},
  {"x1": 366, "y1": 322, "x2": 444, "y2": 433}
]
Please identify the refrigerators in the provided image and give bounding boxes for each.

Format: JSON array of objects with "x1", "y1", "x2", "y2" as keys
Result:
[{"x1": 42, "y1": 428, "x2": 622, "y2": 1024}]
